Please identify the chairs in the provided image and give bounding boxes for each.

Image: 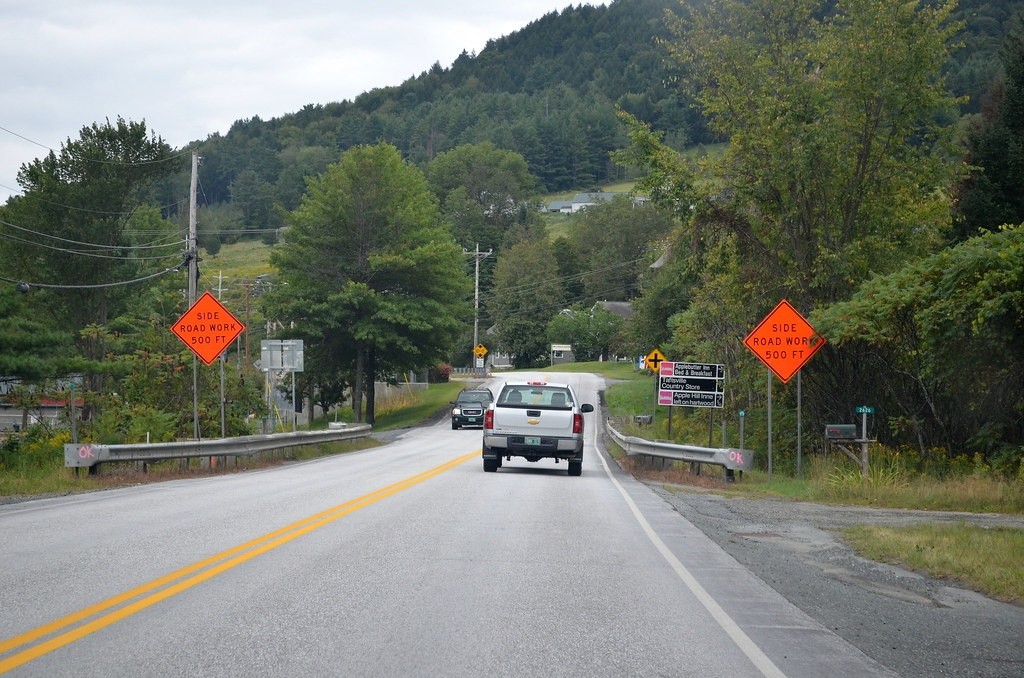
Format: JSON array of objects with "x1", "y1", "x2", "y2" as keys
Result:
[
  {"x1": 507, "y1": 392, "x2": 523, "y2": 404},
  {"x1": 551, "y1": 393, "x2": 567, "y2": 406}
]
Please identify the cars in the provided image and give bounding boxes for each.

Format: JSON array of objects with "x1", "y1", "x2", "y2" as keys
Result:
[
  {"x1": 448, "y1": 387, "x2": 495, "y2": 430},
  {"x1": 481, "y1": 380, "x2": 596, "y2": 477}
]
